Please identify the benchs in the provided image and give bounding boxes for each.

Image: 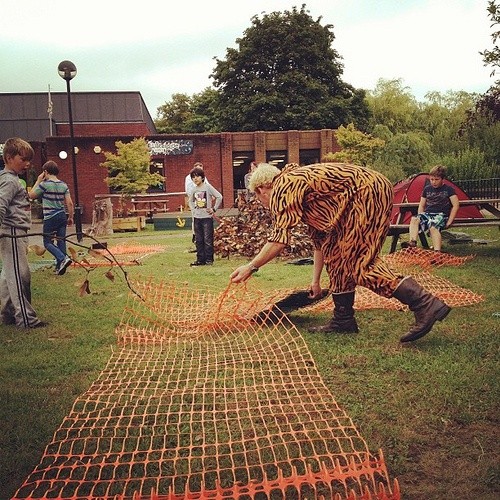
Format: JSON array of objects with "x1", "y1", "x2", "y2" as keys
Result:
[
  {"x1": 128, "y1": 210, "x2": 169, "y2": 213},
  {"x1": 388, "y1": 218, "x2": 500, "y2": 253}
]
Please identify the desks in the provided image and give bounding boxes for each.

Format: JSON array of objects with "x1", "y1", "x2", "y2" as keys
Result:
[
  {"x1": 392, "y1": 199, "x2": 500, "y2": 224},
  {"x1": 132, "y1": 200, "x2": 169, "y2": 209}
]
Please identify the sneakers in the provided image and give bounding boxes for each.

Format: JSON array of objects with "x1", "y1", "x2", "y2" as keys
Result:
[{"x1": 49, "y1": 253, "x2": 77, "y2": 277}]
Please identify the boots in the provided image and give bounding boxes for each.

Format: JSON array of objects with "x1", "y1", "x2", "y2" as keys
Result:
[
  {"x1": 304, "y1": 290, "x2": 363, "y2": 339},
  {"x1": 387, "y1": 275, "x2": 452, "y2": 347}
]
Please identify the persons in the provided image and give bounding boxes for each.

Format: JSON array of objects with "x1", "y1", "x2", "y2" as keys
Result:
[
  {"x1": 184, "y1": 162, "x2": 215, "y2": 244},
  {"x1": 0, "y1": 137, "x2": 48, "y2": 329},
  {"x1": 401, "y1": 164, "x2": 459, "y2": 265},
  {"x1": 229, "y1": 162, "x2": 452, "y2": 342},
  {"x1": 29, "y1": 160, "x2": 73, "y2": 275},
  {"x1": 188, "y1": 168, "x2": 223, "y2": 267}
]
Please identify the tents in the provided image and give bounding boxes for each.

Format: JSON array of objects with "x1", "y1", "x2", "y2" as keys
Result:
[{"x1": 389, "y1": 173, "x2": 484, "y2": 229}]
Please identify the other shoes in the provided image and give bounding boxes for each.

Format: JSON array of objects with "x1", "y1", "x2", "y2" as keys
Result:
[
  {"x1": 32, "y1": 321, "x2": 53, "y2": 330},
  {"x1": 189, "y1": 258, "x2": 215, "y2": 267},
  {"x1": 401, "y1": 238, "x2": 418, "y2": 254}
]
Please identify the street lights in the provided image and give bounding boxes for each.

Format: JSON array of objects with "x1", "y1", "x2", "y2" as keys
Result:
[{"x1": 57, "y1": 60, "x2": 83, "y2": 243}]
